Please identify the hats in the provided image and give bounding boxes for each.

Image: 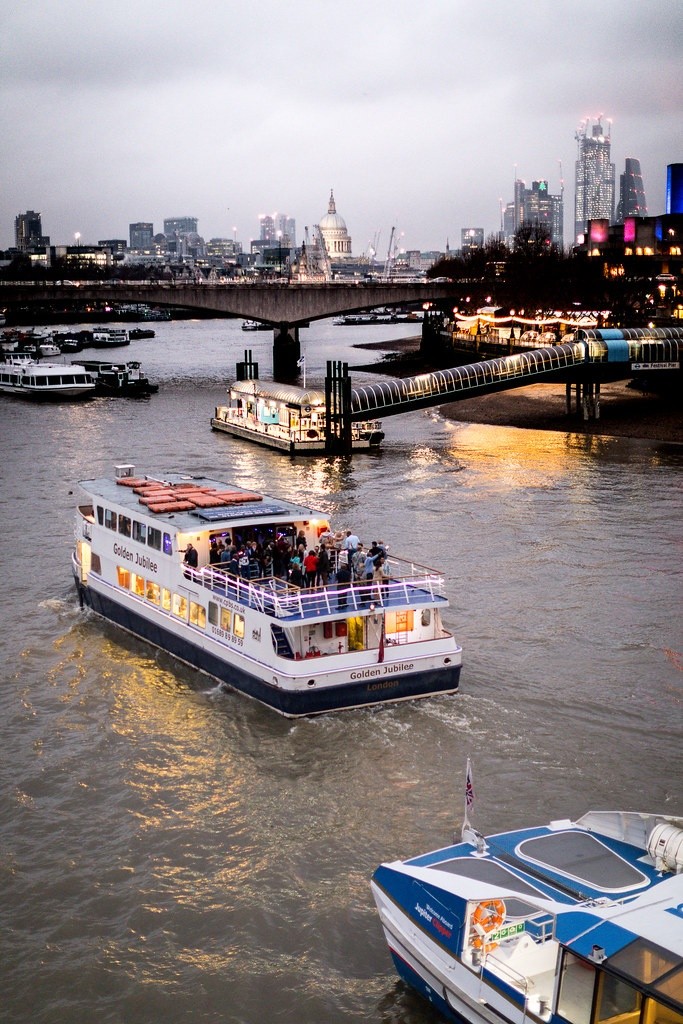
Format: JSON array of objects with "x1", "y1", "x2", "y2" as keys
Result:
[
  {"x1": 335, "y1": 533, "x2": 343, "y2": 539},
  {"x1": 218, "y1": 540, "x2": 222, "y2": 542},
  {"x1": 275, "y1": 533, "x2": 283, "y2": 542}
]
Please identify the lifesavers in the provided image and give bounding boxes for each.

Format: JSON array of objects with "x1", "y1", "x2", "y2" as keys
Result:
[
  {"x1": 474, "y1": 925, "x2": 498, "y2": 952},
  {"x1": 473, "y1": 900, "x2": 507, "y2": 934}
]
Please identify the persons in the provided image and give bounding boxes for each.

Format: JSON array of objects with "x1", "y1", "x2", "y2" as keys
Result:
[
  {"x1": 183, "y1": 543, "x2": 198, "y2": 584},
  {"x1": 209, "y1": 531, "x2": 390, "y2": 611}
]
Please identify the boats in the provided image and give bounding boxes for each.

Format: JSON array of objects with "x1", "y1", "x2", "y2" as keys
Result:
[
  {"x1": 242, "y1": 320, "x2": 274, "y2": 332},
  {"x1": 69, "y1": 464, "x2": 465, "y2": 719},
  {"x1": 370, "y1": 758, "x2": 682, "y2": 1024},
  {"x1": 331, "y1": 305, "x2": 423, "y2": 325},
  {"x1": 0, "y1": 327, "x2": 160, "y2": 400},
  {"x1": 210, "y1": 380, "x2": 385, "y2": 457}
]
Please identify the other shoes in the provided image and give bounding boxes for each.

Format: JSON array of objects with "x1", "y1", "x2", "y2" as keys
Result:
[
  {"x1": 306, "y1": 591, "x2": 309, "y2": 594},
  {"x1": 311, "y1": 591, "x2": 315, "y2": 593},
  {"x1": 335, "y1": 608, "x2": 341, "y2": 610}
]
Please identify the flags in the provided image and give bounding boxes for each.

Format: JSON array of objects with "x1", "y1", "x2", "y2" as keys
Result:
[
  {"x1": 466, "y1": 763, "x2": 475, "y2": 810},
  {"x1": 296, "y1": 354, "x2": 305, "y2": 367}
]
